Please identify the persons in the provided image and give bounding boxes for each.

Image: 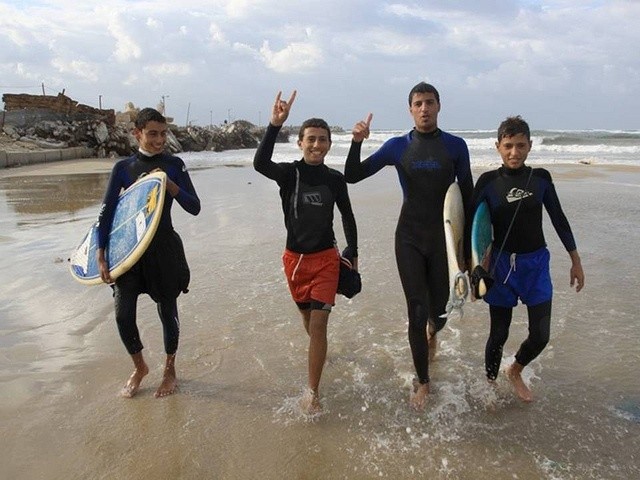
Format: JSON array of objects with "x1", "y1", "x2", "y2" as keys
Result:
[
  {"x1": 252, "y1": 87, "x2": 364, "y2": 416},
  {"x1": 462, "y1": 114, "x2": 585, "y2": 403},
  {"x1": 95, "y1": 106, "x2": 201, "y2": 399},
  {"x1": 342, "y1": 83, "x2": 476, "y2": 410}
]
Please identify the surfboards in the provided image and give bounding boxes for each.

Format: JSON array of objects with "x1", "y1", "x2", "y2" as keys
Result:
[
  {"x1": 471, "y1": 199, "x2": 492, "y2": 300},
  {"x1": 67, "y1": 171, "x2": 167, "y2": 286},
  {"x1": 444, "y1": 182, "x2": 468, "y2": 274}
]
